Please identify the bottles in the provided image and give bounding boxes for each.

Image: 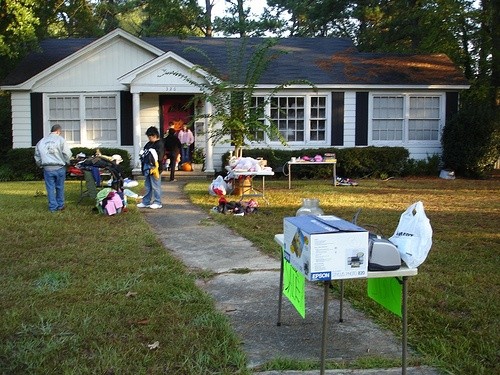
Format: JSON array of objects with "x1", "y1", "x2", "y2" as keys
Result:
[{"x1": 296, "y1": 198, "x2": 324, "y2": 216}]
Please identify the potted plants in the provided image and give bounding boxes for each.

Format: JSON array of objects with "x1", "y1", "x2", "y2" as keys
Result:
[{"x1": 192, "y1": 147, "x2": 205, "y2": 172}]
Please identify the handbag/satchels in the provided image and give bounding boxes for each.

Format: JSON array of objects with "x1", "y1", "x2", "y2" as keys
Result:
[
  {"x1": 389, "y1": 201, "x2": 433, "y2": 267},
  {"x1": 208, "y1": 175, "x2": 229, "y2": 196}
]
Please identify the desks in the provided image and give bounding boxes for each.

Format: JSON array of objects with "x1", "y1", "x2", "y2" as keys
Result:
[
  {"x1": 288, "y1": 161, "x2": 339, "y2": 188},
  {"x1": 225, "y1": 166, "x2": 275, "y2": 207},
  {"x1": 275, "y1": 233, "x2": 418, "y2": 375}
]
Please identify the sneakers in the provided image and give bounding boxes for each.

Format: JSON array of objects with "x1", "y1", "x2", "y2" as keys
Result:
[
  {"x1": 137, "y1": 203, "x2": 150, "y2": 208},
  {"x1": 150, "y1": 204, "x2": 163, "y2": 209}
]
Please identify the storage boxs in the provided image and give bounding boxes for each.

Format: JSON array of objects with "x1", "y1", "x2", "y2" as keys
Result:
[{"x1": 283, "y1": 213, "x2": 369, "y2": 281}]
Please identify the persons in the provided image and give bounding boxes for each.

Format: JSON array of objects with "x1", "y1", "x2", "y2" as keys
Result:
[
  {"x1": 164, "y1": 122, "x2": 175, "y2": 138},
  {"x1": 34, "y1": 124, "x2": 72, "y2": 214},
  {"x1": 136, "y1": 126, "x2": 164, "y2": 209},
  {"x1": 162, "y1": 128, "x2": 181, "y2": 182},
  {"x1": 177, "y1": 123, "x2": 195, "y2": 163}
]
